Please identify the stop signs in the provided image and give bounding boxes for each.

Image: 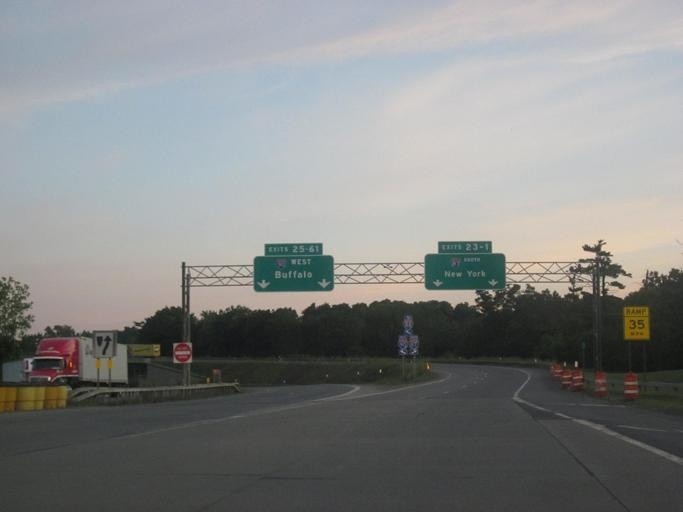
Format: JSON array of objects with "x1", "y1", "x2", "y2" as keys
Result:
[{"x1": 172, "y1": 342, "x2": 193, "y2": 364}]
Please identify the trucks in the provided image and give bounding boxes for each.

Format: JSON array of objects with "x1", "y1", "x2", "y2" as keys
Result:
[{"x1": 21, "y1": 337, "x2": 128, "y2": 398}]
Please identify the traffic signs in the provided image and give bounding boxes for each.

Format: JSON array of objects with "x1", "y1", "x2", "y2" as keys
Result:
[
  {"x1": 254, "y1": 243, "x2": 334, "y2": 292},
  {"x1": 424, "y1": 241, "x2": 506, "y2": 290}
]
